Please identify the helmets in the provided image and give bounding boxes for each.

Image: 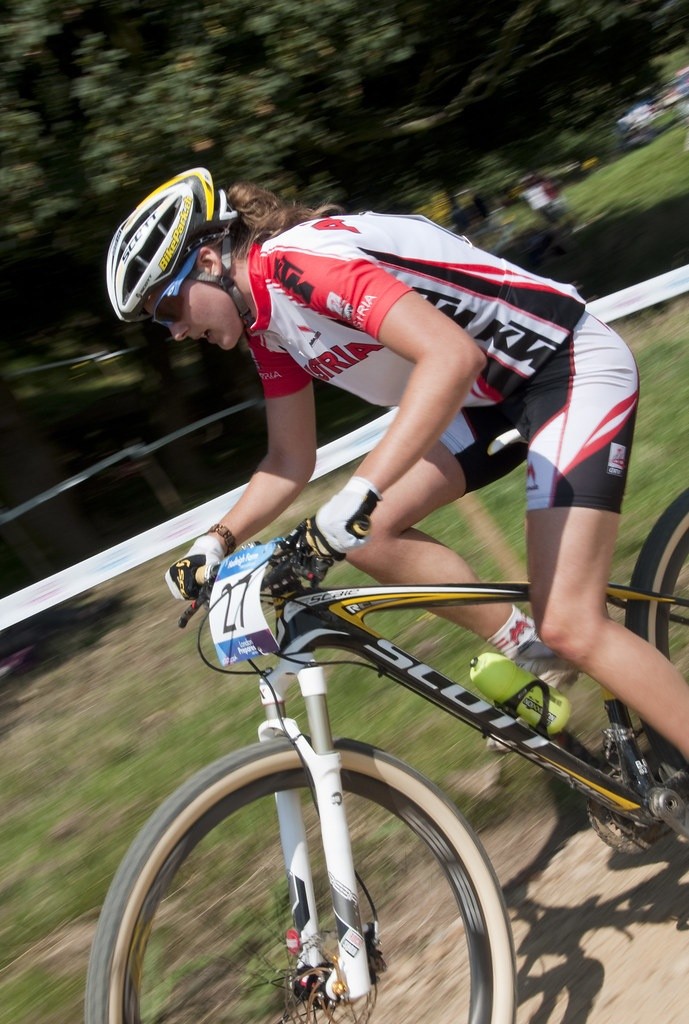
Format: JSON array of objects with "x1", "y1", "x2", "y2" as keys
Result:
[{"x1": 105, "y1": 166, "x2": 239, "y2": 322}]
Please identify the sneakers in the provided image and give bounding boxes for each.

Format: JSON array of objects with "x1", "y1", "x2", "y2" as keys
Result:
[{"x1": 486, "y1": 655, "x2": 583, "y2": 753}]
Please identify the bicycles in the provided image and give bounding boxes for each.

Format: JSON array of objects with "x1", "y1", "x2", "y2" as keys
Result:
[{"x1": 80, "y1": 483, "x2": 689, "y2": 1023}]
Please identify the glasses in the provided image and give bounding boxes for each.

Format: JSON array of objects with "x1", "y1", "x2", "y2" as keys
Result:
[{"x1": 152, "y1": 247, "x2": 205, "y2": 327}]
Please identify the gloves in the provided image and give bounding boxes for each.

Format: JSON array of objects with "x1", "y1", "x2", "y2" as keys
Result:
[
  {"x1": 165, "y1": 536, "x2": 225, "y2": 601},
  {"x1": 306, "y1": 476, "x2": 383, "y2": 562}
]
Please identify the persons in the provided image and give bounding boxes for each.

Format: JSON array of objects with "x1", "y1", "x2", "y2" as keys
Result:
[
  {"x1": 105, "y1": 167, "x2": 689, "y2": 757},
  {"x1": 519, "y1": 175, "x2": 567, "y2": 223}
]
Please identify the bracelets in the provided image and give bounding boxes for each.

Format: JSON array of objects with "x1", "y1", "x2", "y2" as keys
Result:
[{"x1": 209, "y1": 524, "x2": 236, "y2": 556}]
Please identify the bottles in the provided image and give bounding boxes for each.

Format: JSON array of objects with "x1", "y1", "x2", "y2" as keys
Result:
[{"x1": 469, "y1": 652, "x2": 571, "y2": 735}]
